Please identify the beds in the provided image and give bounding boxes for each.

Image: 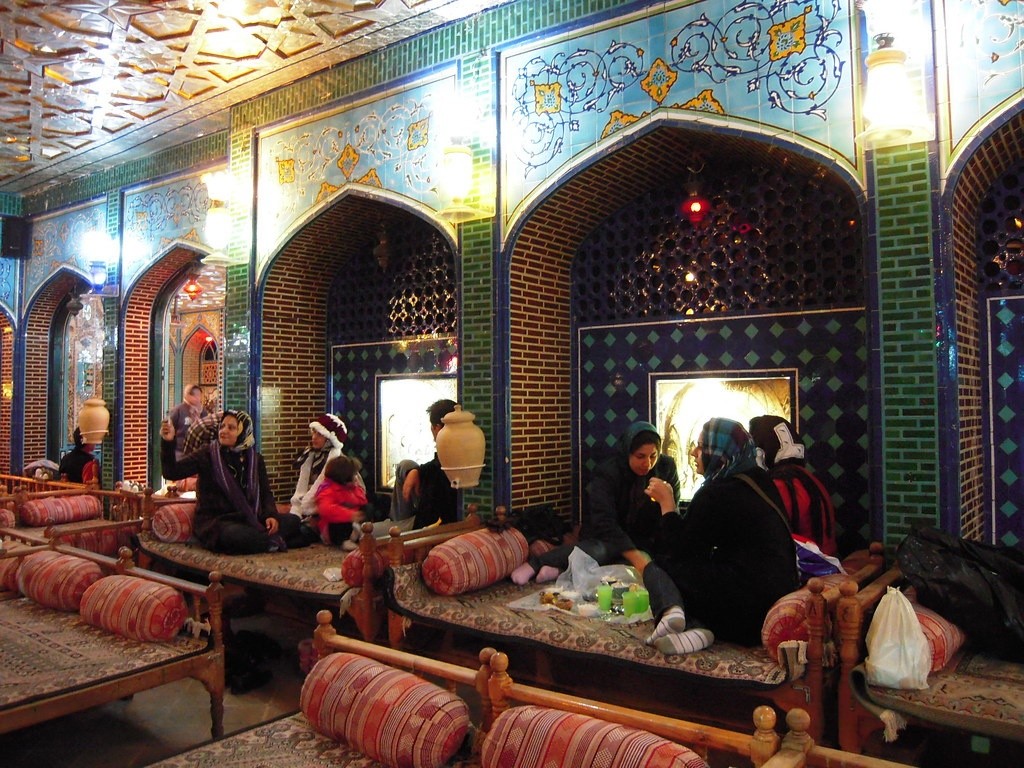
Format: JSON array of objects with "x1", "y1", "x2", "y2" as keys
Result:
[{"x1": 1, "y1": 474, "x2": 1024, "y2": 768}]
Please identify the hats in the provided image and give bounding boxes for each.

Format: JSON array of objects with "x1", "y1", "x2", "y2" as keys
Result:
[{"x1": 309, "y1": 413, "x2": 348, "y2": 450}]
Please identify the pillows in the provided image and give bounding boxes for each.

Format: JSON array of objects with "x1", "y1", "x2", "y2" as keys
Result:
[
  {"x1": 301, "y1": 652, "x2": 468, "y2": 768},
  {"x1": 1, "y1": 509, "x2": 16, "y2": 532},
  {"x1": 0, "y1": 541, "x2": 36, "y2": 591},
  {"x1": 422, "y1": 523, "x2": 531, "y2": 595},
  {"x1": 15, "y1": 550, "x2": 102, "y2": 612},
  {"x1": 760, "y1": 546, "x2": 871, "y2": 661},
  {"x1": 19, "y1": 495, "x2": 102, "y2": 524},
  {"x1": 903, "y1": 585, "x2": 966, "y2": 674},
  {"x1": 80, "y1": 575, "x2": 187, "y2": 643},
  {"x1": 480, "y1": 706, "x2": 710, "y2": 767},
  {"x1": 341, "y1": 542, "x2": 426, "y2": 588},
  {"x1": 48, "y1": 518, "x2": 139, "y2": 555},
  {"x1": 151, "y1": 502, "x2": 201, "y2": 542},
  {"x1": 531, "y1": 525, "x2": 581, "y2": 557}
]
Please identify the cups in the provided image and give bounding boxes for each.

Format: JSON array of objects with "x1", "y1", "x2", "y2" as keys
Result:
[
  {"x1": 599, "y1": 585, "x2": 612, "y2": 610},
  {"x1": 611, "y1": 579, "x2": 629, "y2": 604},
  {"x1": 622, "y1": 590, "x2": 648, "y2": 616}
]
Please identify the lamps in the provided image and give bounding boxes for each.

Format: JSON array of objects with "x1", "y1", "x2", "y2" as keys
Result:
[
  {"x1": 375, "y1": 219, "x2": 394, "y2": 269},
  {"x1": 66, "y1": 284, "x2": 83, "y2": 317},
  {"x1": 433, "y1": 144, "x2": 477, "y2": 224},
  {"x1": 681, "y1": 156, "x2": 709, "y2": 223},
  {"x1": 89, "y1": 261, "x2": 107, "y2": 294},
  {"x1": 200, "y1": 199, "x2": 234, "y2": 267},
  {"x1": 855, "y1": 33, "x2": 915, "y2": 150},
  {"x1": 184, "y1": 271, "x2": 204, "y2": 301}
]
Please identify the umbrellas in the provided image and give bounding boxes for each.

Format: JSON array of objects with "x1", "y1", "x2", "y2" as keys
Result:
[{"x1": 24, "y1": 457, "x2": 60, "y2": 473}]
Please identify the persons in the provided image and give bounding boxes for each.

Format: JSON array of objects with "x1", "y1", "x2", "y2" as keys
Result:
[
  {"x1": 642, "y1": 420, "x2": 801, "y2": 655},
  {"x1": 748, "y1": 415, "x2": 838, "y2": 558},
  {"x1": 510, "y1": 420, "x2": 679, "y2": 588},
  {"x1": 288, "y1": 411, "x2": 366, "y2": 544},
  {"x1": 32, "y1": 466, "x2": 51, "y2": 480},
  {"x1": 169, "y1": 382, "x2": 216, "y2": 461},
  {"x1": 159, "y1": 408, "x2": 303, "y2": 557},
  {"x1": 58, "y1": 423, "x2": 100, "y2": 491},
  {"x1": 318, "y1": 460, "x2": 371, "y2": 547},
  {"x1": 389, "y1": 398, "x2": 460, "y2": 530}
]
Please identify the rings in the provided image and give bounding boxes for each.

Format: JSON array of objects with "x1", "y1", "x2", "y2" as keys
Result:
[{"x1": 647, "y1": 486, "x2": 649, "y2": 489}]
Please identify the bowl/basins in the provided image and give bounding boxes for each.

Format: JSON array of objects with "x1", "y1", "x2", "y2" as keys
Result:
[
  {"x1": 539, "y1": 591, "x2": 574, "y2": 611},
  {"x1": 576, "y1": 604, "x2": 597, "y2": 615}
]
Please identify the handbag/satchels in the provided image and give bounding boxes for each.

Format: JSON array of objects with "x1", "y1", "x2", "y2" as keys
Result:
[
  {"x1": 791, "y1": 533, "x2": 848, "y2": 584},
  {"x1": 864, "y1": 586, "x2": 932, "y2": 688}
]
